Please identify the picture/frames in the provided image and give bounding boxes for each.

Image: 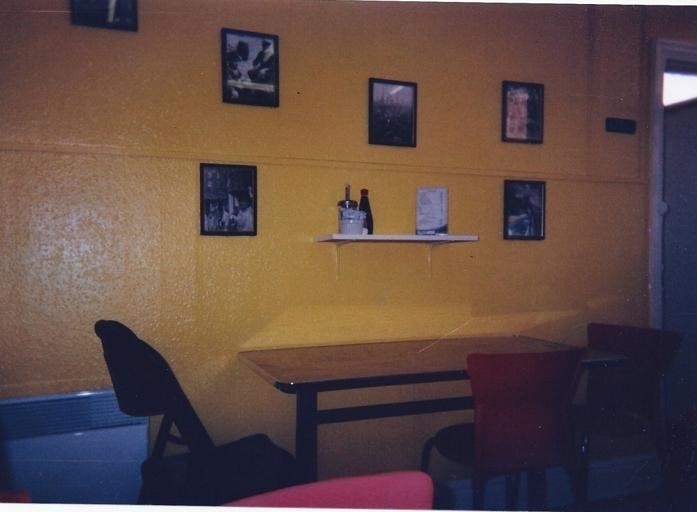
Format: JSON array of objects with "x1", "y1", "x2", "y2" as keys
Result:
[
  {"x1": 222, "y1": 28, "x2": 279, "y2": 109},
  {"x1": 200, "y1": 162, "x2": 258, "y2": 236},
  {"x1": 504, "y1": 179, "x2": 545, "y2": 239},
  {"x1": 70, "y1": 0, "x2": 138, "y2": 33},
  {"x1": 368, "y1": 77, "x2": 417, "y2": 148},
  {"x1": 502, "y1": 79, "x2": 544, "y2": 143}
]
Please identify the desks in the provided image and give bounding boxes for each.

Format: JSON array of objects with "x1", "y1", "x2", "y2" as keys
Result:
[{"x1": 238, "y1": 337, "x2": 586, "y2": 481}]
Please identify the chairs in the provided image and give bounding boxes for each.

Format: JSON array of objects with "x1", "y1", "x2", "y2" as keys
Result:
[
  {"x1": 570, "y1": 322, "x2": 683, "y2": 512},
  {"x1": 219, "y1": 469, "x2": 434, "y2": 507},
  {"x1": 95, "y1": 319, "x2": 311, "y2": 503},
  {"x1": 421, "y1": 349, "x2": 587, "y2": 510}
]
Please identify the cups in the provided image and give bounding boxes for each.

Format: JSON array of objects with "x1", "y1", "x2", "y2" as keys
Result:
[{"x1": 339, "y1": 219, "x2": 364, "y2": 234}]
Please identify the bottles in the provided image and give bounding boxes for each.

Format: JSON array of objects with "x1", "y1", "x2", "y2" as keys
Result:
[
  {"x1": 359, "y1": 188, "x2": 374, "y2": 235},
  {"x1": 337, "y1": 200, "x2": 358, "y2": 220}
]
